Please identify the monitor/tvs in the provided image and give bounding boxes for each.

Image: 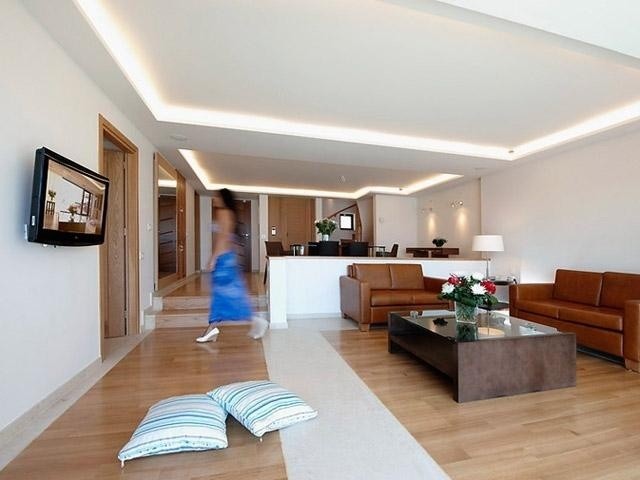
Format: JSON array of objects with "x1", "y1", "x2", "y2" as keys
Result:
[
  {"x1": 25, "y1": 147, "x2": 110, "y2": 248},
  {"x1": 339, "y1": 213, "x2": 355, "y2": 230}
]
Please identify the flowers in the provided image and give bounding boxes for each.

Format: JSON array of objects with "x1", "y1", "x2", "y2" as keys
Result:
[
  {"x1": 68, "y1": 204, "x2": 79, "y2": 214},
  {"x1": 313, "y1": 218, "x2": 338, "y2": 236},
  {"x1": 436, "y1": 272, "x2": 498, "y2": 319}
]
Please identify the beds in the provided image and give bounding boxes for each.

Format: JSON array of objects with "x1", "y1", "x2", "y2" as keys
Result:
[{"x1": 86, "y1": 219, "x2": 100, "y2": 233}]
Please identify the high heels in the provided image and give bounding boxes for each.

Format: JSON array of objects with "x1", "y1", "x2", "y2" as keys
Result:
[{"x1": 196, "y1": 328, "x2": 219, "y2": 342}]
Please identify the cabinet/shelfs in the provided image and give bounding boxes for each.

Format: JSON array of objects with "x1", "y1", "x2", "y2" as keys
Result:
[
  {"x1": 57, "y1": 210, "x2": 87, "y2": 233},
  {"x1": 45, "y1": 200, "x2": 56, "y2": 214}
]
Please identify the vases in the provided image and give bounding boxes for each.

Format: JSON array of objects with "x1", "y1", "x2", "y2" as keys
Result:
[
  {"x1": 322, "y1": 234, "x2": 329, "y2": 241},
  {"x1": 453, "y1": 302, "x2": 479, "y2": 324}
]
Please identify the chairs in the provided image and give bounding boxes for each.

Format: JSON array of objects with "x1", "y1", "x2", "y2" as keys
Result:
[
  {"x1": 264, "y1": 241, "x2": 294, "y2": 285},
  {"x1": 289, "y1": 238, "x2": 400, "y2": 257}
]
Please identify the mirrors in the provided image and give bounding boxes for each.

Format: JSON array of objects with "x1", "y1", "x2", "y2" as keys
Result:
[{"x1": 154, "y1": 151, "x2": 180, "y2": 292}]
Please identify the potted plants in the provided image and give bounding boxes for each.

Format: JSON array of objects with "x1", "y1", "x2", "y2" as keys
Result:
[
  {"x1": 47, "y1": 189, "x2": 58, "y2": 201},
  {"x1": 432, "y1": 238, "x2": 448, "y2": 248}
]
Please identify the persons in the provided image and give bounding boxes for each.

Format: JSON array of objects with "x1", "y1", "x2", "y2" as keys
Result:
[{"x1": 196, "y1": 187, "x2": 269, "y2": 343}]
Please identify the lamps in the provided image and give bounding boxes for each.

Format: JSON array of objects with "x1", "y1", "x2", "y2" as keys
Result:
[
  {"x1": 450, "y1": 200, "x2": 464, "y2": 210},
  {"x1": 421, "y1": 208, "x2": 434, "y2": 215},
  {"x1": 472, "y1": 234, "x2": 505, "y2": 282}
]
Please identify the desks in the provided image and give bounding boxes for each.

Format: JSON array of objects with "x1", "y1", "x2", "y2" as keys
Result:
[
  {"x1": 478, "y1": 281, "x2": 512, "y2": 312},
  {"x1": 406, "y1": 248, "x2": 459, "y2": 258}
]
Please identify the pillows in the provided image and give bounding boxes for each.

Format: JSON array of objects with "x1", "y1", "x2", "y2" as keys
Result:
[
  {"x1": 117, "y1": 393, "x2": 230, "y2": 469},
  {"x1": 206, "y1": 379, "x2": 318, "y2": 442}
]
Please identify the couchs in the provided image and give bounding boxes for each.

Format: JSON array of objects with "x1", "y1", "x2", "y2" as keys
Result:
[
  {"x1": 339, "y1": 263, "x2": 455, "y2": 332},
  {"x1": 508, "y1": 268, "x2": 640, "y2": 373}
]
